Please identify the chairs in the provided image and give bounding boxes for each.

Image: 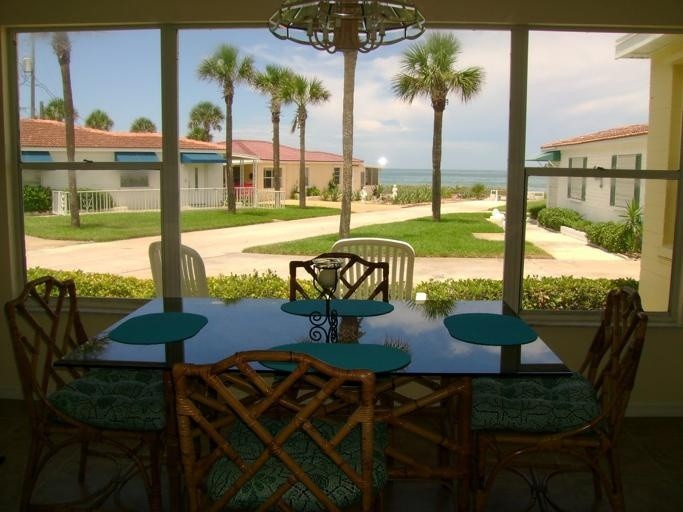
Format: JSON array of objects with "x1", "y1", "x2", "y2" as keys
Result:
[
  {"x1": 149, "y1": 242, "x2": 211, "y2": 299},
  {"x1": 473, "y1": 286, "x2": 650, "y2": 511},
  {"x1": 172, "y1": 352, "x2": 385, "y2": 511},
  {"x1": 4, "y1": 276, "x2": 167, "y2": 512},
  {"x1": 289, "y1": 252, "x2": 389, "y2": 303},
  {"x1": 331, "y1": 237, "x2": 414, "y2": 301}
]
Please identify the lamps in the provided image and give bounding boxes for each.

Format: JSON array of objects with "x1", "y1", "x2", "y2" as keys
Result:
[{"x1": 269, "y1": 1, "x2": 427, "y2": 54}]
